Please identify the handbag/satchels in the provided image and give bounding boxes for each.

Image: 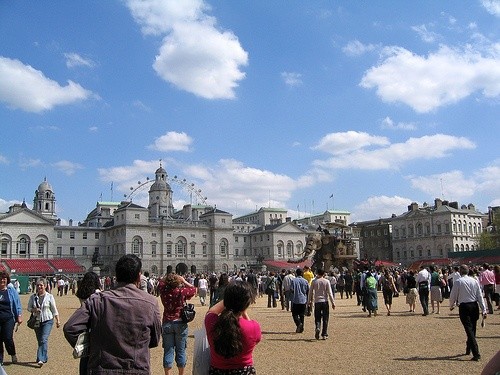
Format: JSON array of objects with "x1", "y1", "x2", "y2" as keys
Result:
[
  {"x1": 439, "y1": 278, "x2": 446, "y2": 287},
  {"x1": 192, "y1": 323, "x2": 210, "y2": 375},
  {"x1": 27, "y1": 312, "x2": 40, "y2": 328},
  {"x1": 305, "y1": 303, "x2": 312, "y2": 316},
  {"x1": 393, "y1": 291, "x2": 399, "y2": 297},
  {"x1": 181, "y1": 304, "x2": 196, "y2": 323}
]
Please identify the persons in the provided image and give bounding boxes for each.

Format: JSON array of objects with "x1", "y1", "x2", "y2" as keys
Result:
[
  {"x1": 63, "y1": 254, "x2": 162, "y2": 375},
  {"x1": 160, "y1": 274, "x2": 196, "y2": 375},
  {"x1": 289, "y1": 267, "x2": 336, "y2": 340},
  {"x1": 448, "y1": 264, "x2": 487, "y2": 360},
  {"x1": 0, "y1": 271, "x2": 23, "y2": 367},
  {"x1": 32, "y1": 262, "x2": 500, "y2": 318},
  {"x1": 204, "y1": 284, "x2": 262, "y2": 375},
  {"x1": 27, "y1": 278, "x2": 61, "y2": 366}
]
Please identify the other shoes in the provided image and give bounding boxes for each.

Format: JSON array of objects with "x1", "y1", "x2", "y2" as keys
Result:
[
  {"x1": 466, "y1": 343, "x2": 471, "y2": 355},
  {"x1": 362, "y1": 308, "x2": 366, "y2": 312},
  {"x1": 470, "y1": 355, "x2": 481, "y2": 361},
  {"x1": 38, "y1": 360, "x2": 43, "y2": 368},
  {"x1": 296, "y1": 323, "x2": 304, "y2": 333},
  {"x1": 265, "y1": 289, "x2": 268, "y2": 295},
  {"x1": 11, "y1": 355, "x2": 17, "y2": 364},
  {"x1": 315, "y1": 328, "x2": 320, "y2": 340},
  {"x1": 323, "y1": 335, "x2": 329, "y2": 340}
]
